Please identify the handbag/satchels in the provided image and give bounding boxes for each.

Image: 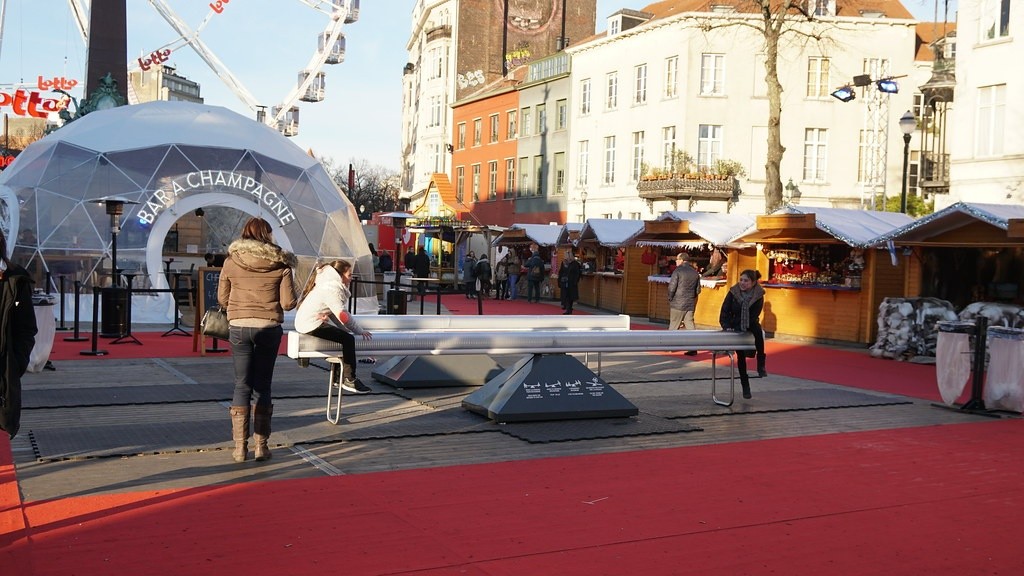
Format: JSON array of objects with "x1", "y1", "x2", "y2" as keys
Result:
[
  {"x1": 405, "y1": 269, "x2": 414, "y2": 276},
  {"x1": 560, "y1": 277, "x2": 569, "y2": 288},
  {"x1": 201, "y1": 306, "x2": 229, "y2": 339},
  {"x1": 515, "y1": 275, "x2": 520, "y2": 283},
  {"x1": 475, "y1": 277, "x2": 481, "y2": 291}
]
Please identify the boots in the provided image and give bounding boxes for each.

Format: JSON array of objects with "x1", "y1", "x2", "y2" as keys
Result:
[
  {"x1": 740, "y1": 375, "x2": 752, "y2": 399},
  {"x1": 229, "y1": 406, "x2": 250, "y2": 462},
  {"x1": 252, "y1": 404, "x2": 274, "y2": 461},
  {"x1": 757, "y1": 354, "x2": 768, "y2": 376}
]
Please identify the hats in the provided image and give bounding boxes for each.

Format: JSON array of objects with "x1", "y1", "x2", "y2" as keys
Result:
[{"x1": 419, "y1": 247, "x2": 426, "y2": 251}]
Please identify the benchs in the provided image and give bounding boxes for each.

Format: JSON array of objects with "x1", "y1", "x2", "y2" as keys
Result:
[{"x1": 429, "y1": 267, "x2": 467, "y2": 295}]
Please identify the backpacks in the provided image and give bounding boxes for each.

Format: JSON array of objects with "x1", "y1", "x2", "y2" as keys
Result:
[{"x1": 531, "y1": 266, "x2": 541, "y2": 281}]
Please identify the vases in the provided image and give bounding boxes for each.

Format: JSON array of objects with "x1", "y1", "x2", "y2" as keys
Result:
[{"x1": 640, "y1": 148, "x2": 752, "y2": 181}]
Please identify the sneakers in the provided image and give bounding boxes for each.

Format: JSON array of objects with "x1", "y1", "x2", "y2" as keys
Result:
[
  {"x1": 342, "y1": 378, "x2": 372, "y2": 394},
  {"x1": 333, "y1": 377, "x2": 340, "y2": 387}
]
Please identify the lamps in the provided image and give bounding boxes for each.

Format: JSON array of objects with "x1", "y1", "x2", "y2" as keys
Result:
[
  {"x1": 195, "y1": 207, "x2": 205, "y2": 218},
  {"x1": 830, "y1": 86, "x2": 856, "y2": 102},
  {"x1": 853, "y1": 74, "x2": 872, "y2": 87},
  {"x1": 876, "y1": 78, "x2": 901, "y2": 94}
]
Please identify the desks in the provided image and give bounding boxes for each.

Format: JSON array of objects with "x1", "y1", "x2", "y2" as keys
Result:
[
  {"x1": 158, "y1": 271, "x2": 195, "y2": 337},
  {"x1": 20, "y1": 252, "x2": 107, "y2": 292},
  {"x1": 102, "y1": 273, "x2": 151, "y2": 345},
  {"x1": 405, "y1": 277, "x2": 440, "y2": 315},
  {"x1": 161, "y1": 259, "x2": 183, "y2": 284},
  {"x1": 74, "y1": 253, "x2": 106, "y2": 294}
]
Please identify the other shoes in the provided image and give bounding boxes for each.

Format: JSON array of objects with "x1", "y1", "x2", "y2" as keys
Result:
[
  {"x1": 536, "y1": 301, "x2": 539, "y2": 303},
  {"x1": 563, "y1": 310, "x2": 572, "y2": 314},
  {"x1": 493, "y1": 297, "x2": 501, "y2": 300},
  {"x1": 528, "y1": 301, "x2": 530, "y2": 303},
  {"x1": 685, "y1": 351, "x2": 697, "y2": 355},
  {"x1": 507, "y1": 297, "x2": 517, "y2": 300},
  {"x1": 562, "y1": 307, "x2": 566, "y2": 309},
  {"x1": 501, "y1": 295, "x2": 504, "y2": 300}
]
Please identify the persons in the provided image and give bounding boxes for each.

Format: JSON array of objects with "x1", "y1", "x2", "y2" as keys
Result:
[
  {"x1": 405, "y1": 246, "x2": 430, "y2": 292},
  {"x1": 369, "y1": 243, "x2": 393, "y2": 273},
  {"x1": 704, "y1": 253, "x2": 726, "y2": 276},
  {"x1": 295, "y1": 259, "x2": 373, "y2": 394},
  {"x1": 667, "y1": 252, "x2": 701, "y2": 356},
  {"x1": 216, "y1": 217, "x2": 297, "y2": 462},
  {"x1": 205, "y1": 253, "x2": 226, "y2": 267},
  {"x1": 719, "y1": 270, "x2": 767, "y2": 400},
  {"x1": 461, "y1": 251, "x2": 492, "y2": 299},
  {"x1": 557, "y1": 251, "x2": 581, "y2": 314},
  {"x1": 0, "y1": 228, "x2": 38, "y2": 441},
  {"x1": 494, "y1": 247, "x2": 521, "y2": 300},
  {"x1": 524, "y1": 251, "x2": 546, "y2": 303}
]
